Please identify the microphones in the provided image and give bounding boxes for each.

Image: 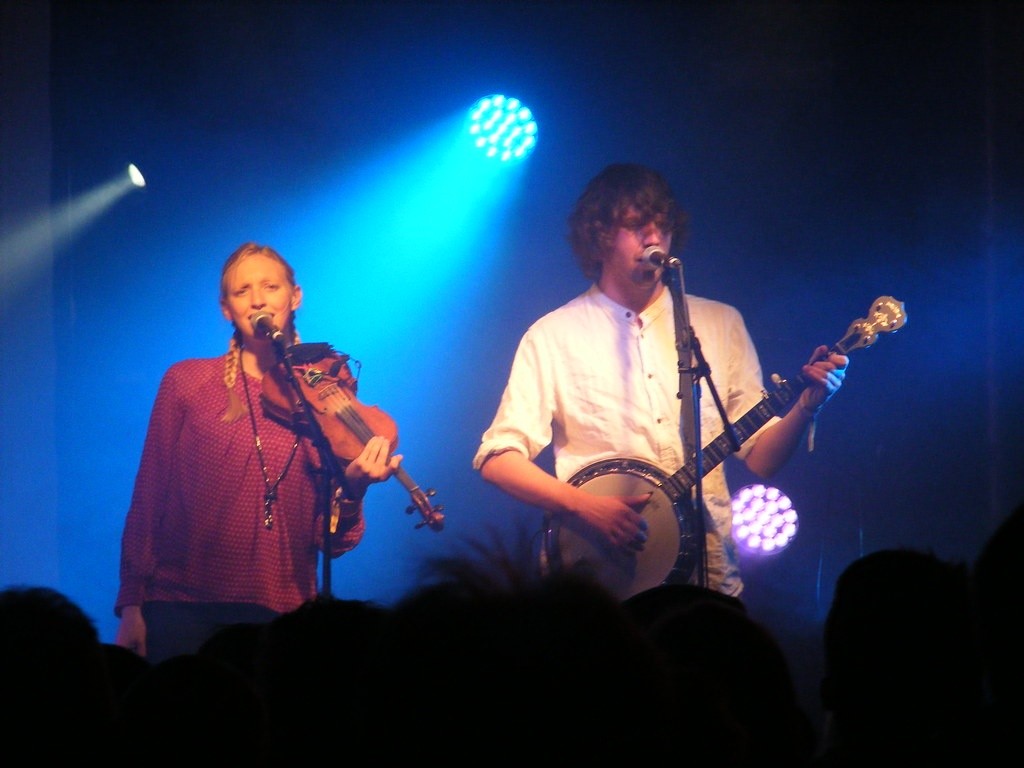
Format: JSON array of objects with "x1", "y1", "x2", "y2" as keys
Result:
[
  {"x1": 249, "y1": 310, "x2": 284, "y2": 341},
  {"x1": 641, "y1": 245, "x2": 682, "y2": 270}
]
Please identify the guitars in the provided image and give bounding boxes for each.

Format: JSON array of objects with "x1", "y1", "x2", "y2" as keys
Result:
[{"x1": 540, "y1": 295, "x2": 909, "y2": 600}]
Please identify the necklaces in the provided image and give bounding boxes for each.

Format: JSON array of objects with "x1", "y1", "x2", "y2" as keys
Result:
[{"x1": 239, "y1": 349, "x2": 303, "y2": 528}]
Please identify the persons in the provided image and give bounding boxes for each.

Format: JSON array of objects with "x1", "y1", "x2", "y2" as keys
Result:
[
  {"x1": 0, "y1": 511, "x2": 1023, "y2": 768},
  {"x1": 114, "y1": 243, "x2": 402, "y2": 656},
  {"x1": 474, "y1": 165, "x2": 849, "y2": 605}
]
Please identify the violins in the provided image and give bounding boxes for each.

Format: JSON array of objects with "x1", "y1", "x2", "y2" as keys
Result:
[{"x1": 258, "y1": 341, "x2": 447, "y2": 533}]
